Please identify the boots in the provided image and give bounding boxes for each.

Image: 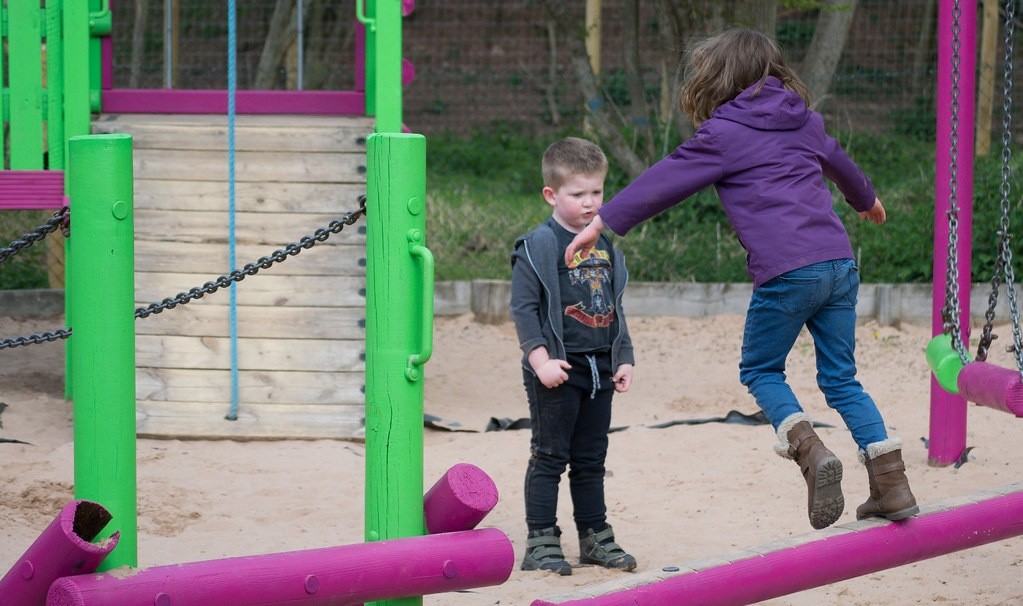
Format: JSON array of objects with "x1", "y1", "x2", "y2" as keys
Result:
[
  {"x1": 774, "y1": 413, "x2": 844, "y2": 530},
  {"x1": 856, "y1": 437, "x2": 919, "y2": 520}
]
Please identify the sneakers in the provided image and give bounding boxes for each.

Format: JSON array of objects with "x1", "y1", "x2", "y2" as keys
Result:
[
  {"x1": 579, "y1": 525, "x2": 636, "y2": 570},
  {"x1": 521, "y1": 526, "x2": 572, "y2": 576}
]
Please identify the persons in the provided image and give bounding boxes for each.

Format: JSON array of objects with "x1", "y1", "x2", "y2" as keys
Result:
[
  {"x1": 512, "y1": 136, "x2": 636, "y2": 575},
  {"x1": 565, "y1": 30, "x2": 920, "y2": 529}
]
